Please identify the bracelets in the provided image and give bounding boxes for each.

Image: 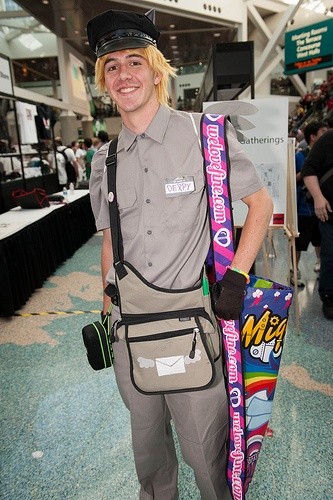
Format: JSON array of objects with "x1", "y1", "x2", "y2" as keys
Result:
[{"x1": 226, "y1": 265, "x2": 250, "y2": 284}]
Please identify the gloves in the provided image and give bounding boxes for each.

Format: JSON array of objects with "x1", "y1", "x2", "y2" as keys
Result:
[
  {"x1": 100, "y1": 310, "x2": 108, "y2": 334},
  {"x1": 209, "y1": 265, "x2": 251, "y2": 321}
]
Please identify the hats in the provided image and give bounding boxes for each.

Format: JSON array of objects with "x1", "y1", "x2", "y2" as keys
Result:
[{"x1": 86, "y1": 9, "x2": 161, "y2": 58}]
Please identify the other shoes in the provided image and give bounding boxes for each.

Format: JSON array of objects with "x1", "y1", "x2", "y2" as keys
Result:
[
  {"x1": 287, "y1": 267, "x2": 304, "y2": 286},
  {"x1": 322, "y1": 301, "x2": 333, "y2": 321},
  {"x1": 314, "y1": 263, "x2": 321, "y2": 272}
]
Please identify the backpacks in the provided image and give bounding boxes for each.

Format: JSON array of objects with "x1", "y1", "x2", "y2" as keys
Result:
[{"x1": 57, "y1": 147, "x2": 78, "y2": 182}]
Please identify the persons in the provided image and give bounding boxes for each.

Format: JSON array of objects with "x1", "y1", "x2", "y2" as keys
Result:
[
  {"x1": 0, "y1": 136, "x2": 49, "y2": 182},
  {"x1": 288, "y1": 79, "x2": 333, "y2": 153},
  {"x1": 301, "y1": 128, "x2": 333, "y2": 321},
  {"x1": 47, "y1": 130, "x2": 109, "y2": 191},
  {"x1": 89, "y1": 9, "x2": 274, "y2": 500},
  {"x1": 288, "y1": 122, "x2": 328, "y2": 287}
]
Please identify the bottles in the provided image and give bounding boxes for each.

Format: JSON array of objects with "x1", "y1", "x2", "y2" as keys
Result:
[
  {"x1": 63, "y1": 187, "x2": 67, "y2": 200},
  {"x1": 69, "y1": 183, "x2": 74, "y2": 195}
]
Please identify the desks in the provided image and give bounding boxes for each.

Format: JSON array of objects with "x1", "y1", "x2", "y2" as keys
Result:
[{"x1": 0, "y1": 189, "x2": 97, "y2": 319}]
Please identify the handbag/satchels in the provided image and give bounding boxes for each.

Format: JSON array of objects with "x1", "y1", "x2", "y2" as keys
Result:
[
  {"x1": 218, "y1": 273, "x2": 293, "y2": 500},
  {"x1": 298, "y1": 183, "x2": 315, "y2": 207},
  {"x1": 81, "y1": 321, "x2": 114, "y2": 371},
  {"x1": 12, "y1": 187, "x2": 51, "y2": 210},
  {"x1": 110, "y1": 260, "x2": 222, "y2": 396}
]
